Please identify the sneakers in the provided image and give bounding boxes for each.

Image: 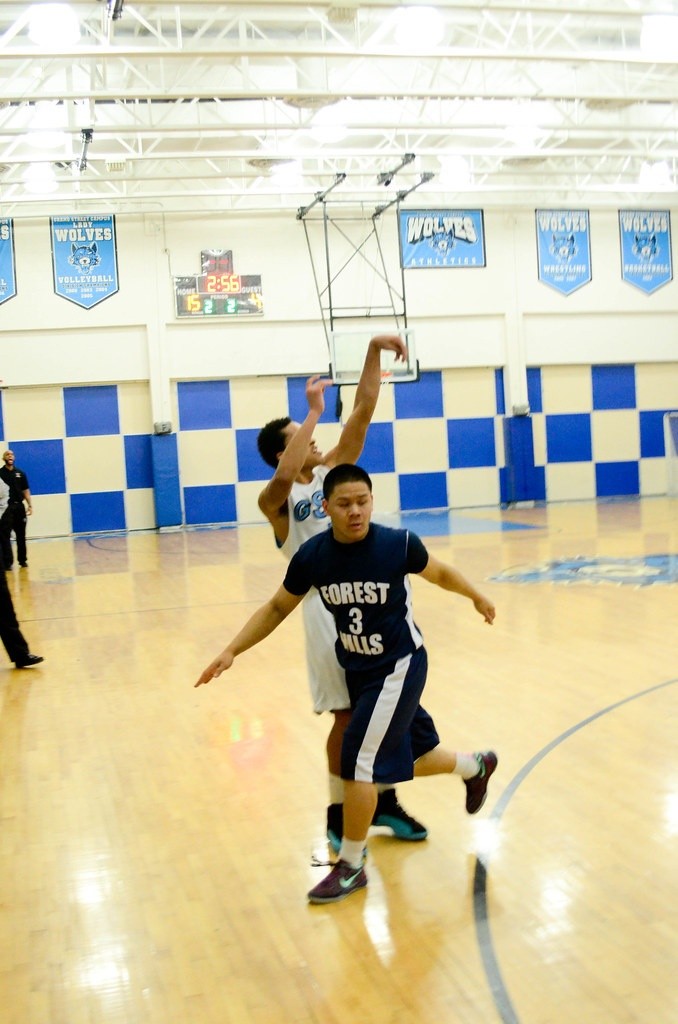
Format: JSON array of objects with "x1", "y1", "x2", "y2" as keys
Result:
[
  {"x1": 307, "y1": 856, "x2": 367, "y2": 904},
  {"x1": 463, "y1": 752, "x2": 498, "y2": 814},
  {"x1": 326, "y1": 803, "x2": 367, "y2": 857},
  {"x1": 369, "y1": 787, "x2": 428, "y2": 840}
]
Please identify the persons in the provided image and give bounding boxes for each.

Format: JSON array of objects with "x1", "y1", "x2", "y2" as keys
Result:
[
  {"x1": 0, "y1": 449, "x2": 34, "y2": 570},
  {"x1": 258, "y1": 335, "x2": 427, "y2": 855},
  {"x1": 195, "y1": 463, "x2": 497, "y2": 902},
  {"x1": 0, "y1": 479, "x2": 44, "y2": 669}
]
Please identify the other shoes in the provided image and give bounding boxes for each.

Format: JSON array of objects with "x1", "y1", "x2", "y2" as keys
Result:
[
  {"x1": 20, "y1": 561, "x2": 28, "y2": 567},
  {"x1": 14, "y1": 653, "x2": 43, "y2": 668}
]
